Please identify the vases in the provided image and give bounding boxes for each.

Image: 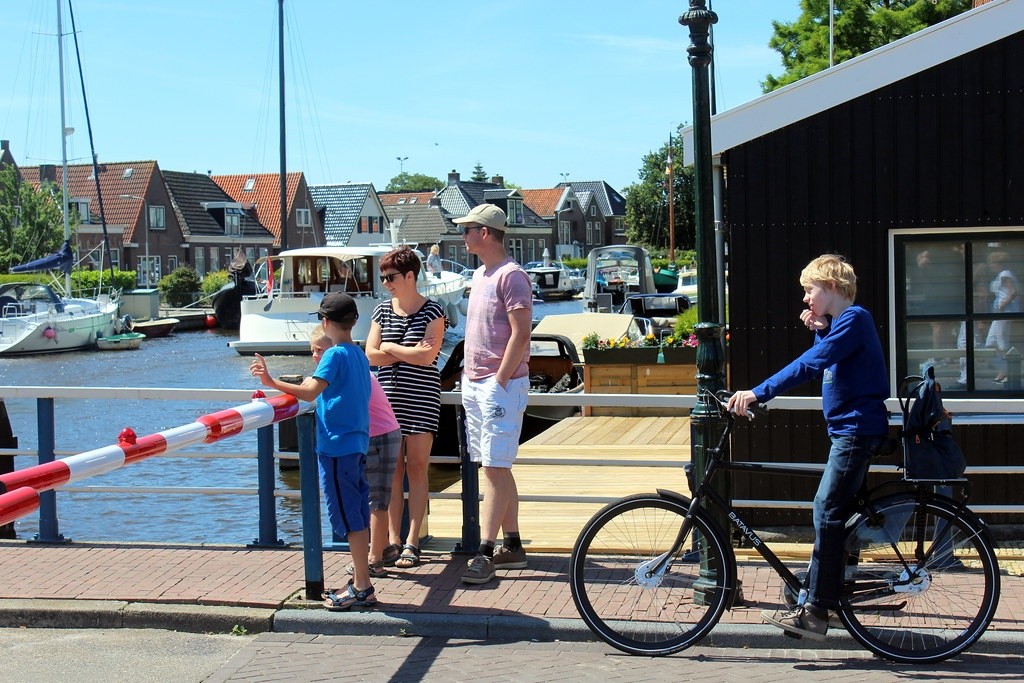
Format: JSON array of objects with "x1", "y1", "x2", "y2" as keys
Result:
[
  {"x1": 583, "y1": 346, "x2": 658, "y2": 364},
  {"x1": 663, "y1": 347, "x2": 729, "y2": 363}
]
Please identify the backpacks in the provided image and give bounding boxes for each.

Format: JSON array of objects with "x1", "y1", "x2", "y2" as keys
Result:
[{"x1": 898, "y1": 365, "x2": 967, "y2": 479}]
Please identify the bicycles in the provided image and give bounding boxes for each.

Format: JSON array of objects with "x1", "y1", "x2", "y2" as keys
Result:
[{"x1": 571, "y1": 388, "x2": 1001, "y2": 665}]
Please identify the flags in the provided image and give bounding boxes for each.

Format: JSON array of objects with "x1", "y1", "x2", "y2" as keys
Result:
[{"x1": 264, "y1": 256, "x2": 273, "y2": 311}]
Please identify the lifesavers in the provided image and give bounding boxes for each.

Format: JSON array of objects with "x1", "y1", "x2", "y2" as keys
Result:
[{"x1": 562, "y1": 279, "x2": 569, "y2": 288}]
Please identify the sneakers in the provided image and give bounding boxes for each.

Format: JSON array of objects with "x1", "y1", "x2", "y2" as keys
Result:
[
  {"x1": 467, "y1": 545, "x2": 528, "y2": 568},
  {"x1": 759, "y1": 602, "x2": 829, "y2": 642},
  {"x1": 461, "y1": 555, "x2": 495, "y2": 584}
]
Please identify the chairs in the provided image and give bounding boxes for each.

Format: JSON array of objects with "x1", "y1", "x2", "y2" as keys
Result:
[
  {"x1": 596, "y1": 293, "x2": 612, "y2": 313},
  {"x1": 625, "y1": 293, "x2": 639, "y2": 301}
]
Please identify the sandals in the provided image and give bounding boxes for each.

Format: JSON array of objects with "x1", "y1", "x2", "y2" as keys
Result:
[
  {"x1": 322, "y1": 584, "x2": 377, "y2": 610},
  {"x1": 321, "y1": 579, "x2": 353, "y2": 599},
  {"x1": 382, "y1": 544, "x2": 400, "y2": 567},
  {"x1": 395, "y1": 544, "x2": 420, "y2": 567},
  {"x1": 346, "y1": 560, "x2": 387, "y2": 578}
]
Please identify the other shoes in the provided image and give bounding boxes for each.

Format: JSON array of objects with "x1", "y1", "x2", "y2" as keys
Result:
[
  {"x1": 944, "y1": 381, "x2": 967, "y2": 390},
  {"x1": 992, "y1": 375, "x2": 1009, "y2": 385}
]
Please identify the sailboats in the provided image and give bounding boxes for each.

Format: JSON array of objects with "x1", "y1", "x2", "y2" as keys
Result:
[{"x1": 0, "y1": 1, "x2": 120, "y2": 355}]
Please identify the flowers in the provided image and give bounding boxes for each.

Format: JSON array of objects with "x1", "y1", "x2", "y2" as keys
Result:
[
  {"x1": 664, "y1": 334, "x2": 730, "y2": 348},
  {"x1": 582, "y1": 331, "x2": 660, "y2": 351}
]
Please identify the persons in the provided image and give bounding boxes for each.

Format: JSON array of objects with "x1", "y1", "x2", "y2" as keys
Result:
[
  {"x1": 452, "y1": 204, "x2": 533, "y2": 582},
  {"x1": 249, "y1": 292, "x2": 378, "y2": 611},
  {"x1": 910, "y1": 248, "x2": 1020, "y2": 391},
  {"x1": 427, "y1": 244, "x2": 442, "y2": 279},
  {"x1": 365, "y1": 246, "x2": 449, "y2": 566},
  {"x1": 308, "y1": 325, "x2": 401, "y2": 578},
  {"x1": 726, "y1": 254, "x2": 891, "y2": 640}
]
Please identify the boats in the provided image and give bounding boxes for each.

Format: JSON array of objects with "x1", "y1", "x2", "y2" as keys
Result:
[
  {"x1": 619, "y1": 294, "x2": 693, "y2": 338},
  {"x1": 667, "y1": 269, "x2": 730, "y2": 304},
  {"x1": 459, "y1": 269, "x2": 475, "y2": 298},
  {"x1": 127, "y1": 318, "x2": 181, "y2": 338},
  {"x1": 226, "y1": 245, "x2": 465, "y2": 354},
  {"x1": 525, "y1": 267, "x2": 585, "y2": 301},
  {"x1": 151, "y1": 308, "x2": 208, "y2": 331},
  {"x1": 505, "y1": 312, "x2": 655, "y2": 428},
  {"x1": 96, "y1": 332, "x2": 147, "y2": 350},
  {"x1": 584, "y1": 244, "x2": 657, "y2": 312}
]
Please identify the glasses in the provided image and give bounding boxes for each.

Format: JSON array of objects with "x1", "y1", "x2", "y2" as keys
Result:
[
  {"x1": 318, "y1": 313, "x2": 324, "y2": 321},
  {"x1": 379, "y1": 272, "x2": 402, "y2": 283},
  {"x1": 464, "y1": 225, "x2": 480, "y2": 235}
]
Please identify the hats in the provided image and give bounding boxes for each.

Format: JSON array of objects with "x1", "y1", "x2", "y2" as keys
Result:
[
  {"x1": 309, "y1": 291, "x2": 359, "y2": 320},
  {"x1": 452, "y1": 203, "x2": 508, "y2": 232}
]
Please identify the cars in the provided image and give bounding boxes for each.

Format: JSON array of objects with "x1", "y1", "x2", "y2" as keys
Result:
[{"x1": 523, "y1": 261, "x2": 580, "y2": 276}]
